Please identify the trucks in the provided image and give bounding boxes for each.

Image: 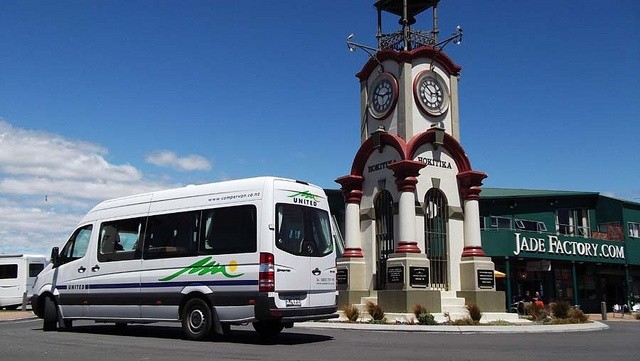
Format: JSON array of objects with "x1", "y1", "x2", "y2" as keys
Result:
[{"x1": 0, "y1": 255, "x2": 42, "y2": 307}]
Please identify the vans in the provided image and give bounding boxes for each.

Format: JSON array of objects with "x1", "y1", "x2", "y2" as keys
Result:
[{"x1": 32, "y1": 177, "x2": 340, "y2": 338}]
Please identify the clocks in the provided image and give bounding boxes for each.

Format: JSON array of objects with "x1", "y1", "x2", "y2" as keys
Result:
[
  {"x1": 367, "y1": 72, "x2": 399, "y2": 120},
  {"x1": 413, "y1": 69, "x2": 450, "y2": 117}
]
